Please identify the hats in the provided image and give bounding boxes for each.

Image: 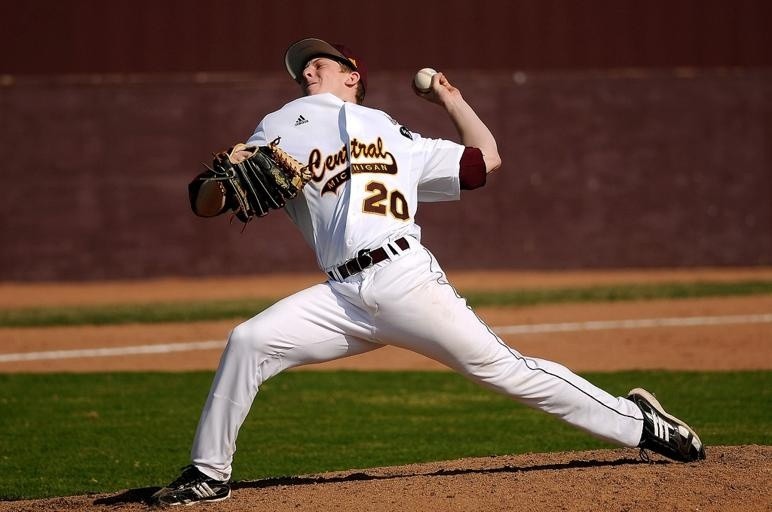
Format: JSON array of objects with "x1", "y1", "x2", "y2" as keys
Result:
[{"x1": 285, "y1": 37, "x2": 367, "y2": 99}]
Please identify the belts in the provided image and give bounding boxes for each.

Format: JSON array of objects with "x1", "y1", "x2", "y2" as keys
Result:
[{"x1": 328, "y1": 237, "x2": 409, "y2": 281}]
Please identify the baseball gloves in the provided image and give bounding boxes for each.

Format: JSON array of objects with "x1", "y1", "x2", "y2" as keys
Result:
[{"x1": 190, "y1": 144, "x2": 313, "y2": 222}]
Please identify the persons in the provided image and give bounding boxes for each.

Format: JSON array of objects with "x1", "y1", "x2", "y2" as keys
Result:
[{"x1": 150, "y1": 36, "x2": 708, "y2": 509}]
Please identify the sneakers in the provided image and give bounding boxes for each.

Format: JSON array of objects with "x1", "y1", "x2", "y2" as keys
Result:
[
  {"x1": 625, "y1": 387, "x2": 705, "y2": 463},
  {"x1": 157, "y1": 464, "x2": 231, "y2": 506}
]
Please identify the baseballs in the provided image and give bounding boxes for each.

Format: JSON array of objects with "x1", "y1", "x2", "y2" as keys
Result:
[{"x1": 414, "y1": 66, "x2": 439, "y2": 94}]
